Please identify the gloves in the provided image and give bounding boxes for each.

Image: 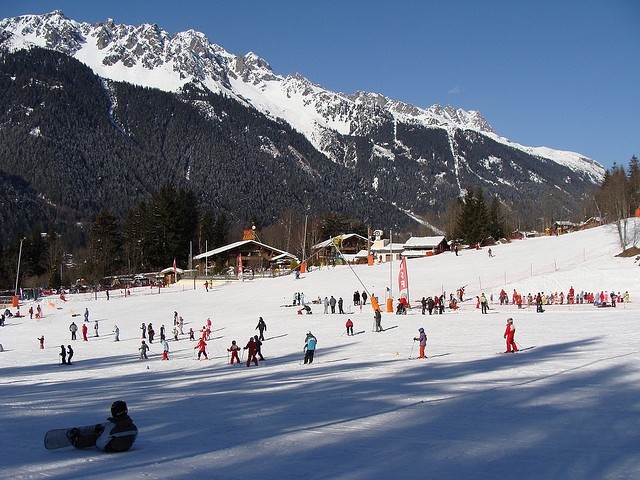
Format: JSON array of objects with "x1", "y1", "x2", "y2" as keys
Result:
[{"x1": 414, "y1": 338, "x2": 417, "y2": 340}]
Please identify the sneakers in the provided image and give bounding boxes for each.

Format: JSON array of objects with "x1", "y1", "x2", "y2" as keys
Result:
[
  {"x1": 95, "y1": 424, "x2": 103, "y2": 433},
  {"x1": 68, "y1": 430, "x2": 81, "y2": 447}
]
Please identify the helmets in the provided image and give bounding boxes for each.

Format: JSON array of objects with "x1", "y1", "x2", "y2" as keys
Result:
[
  {"x1": 232, "y1": 341, "x2": 236, "y2": 345},
  {"x1": 111, "y1": 401, "x2": 128, "y2": 418},
  {"x1": 419, "y1": 328, "x2": 424, "y2": 333},
  {"x1": 507, "y1": 318, "x2": 513, "y2": 322}
]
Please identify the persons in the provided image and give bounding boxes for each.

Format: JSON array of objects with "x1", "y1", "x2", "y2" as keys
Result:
[
  {"x1": 243, "y1": 335, "x2": 258, "y2": 366},
  {"x1": 161, "y1": 338, "x2": 170, "y2": 361},
  {"x1": 93, "y1": 317, "x2": 99, "y2": 337},
  {"x1": 487, "y1": 247, "x2": 494, "y2": 259},
  {"x1": 171, "y1": 326, "x2": 180, "y2": 340},
  {"x1": 210, "y1": 278, "x2": 214, "y2": 290},
  {"x1": 225, "y1": 339, "x2": 241, "y2": 364},
  {"x1": 112, "y1": 323, "x2": 120, "y2": 342},
  {"x1": 36, "y1": 304, "x2": 43, "y2": 317},
  {"x1": 536, "y1": 292, "x2": 543, "y2": 312},
  {"x1": 344, "y1": 319, "x2": 357, "y2": 336},
  {"x1": 455, "y1": 287, "x2": 467, "y2": 302},
  {"x1": 298, "y1": 290, "x2": 301, "y2": 304},
  {"x1": 173, "y1": 310, "x2": 179, "y2": 324},
  {"x1": 322, "y1": 296, "x2": 328, "y2": 315},
  {"x1": 301, "y1": 302, "x2": 312, "y2": 315},
  {"x1": 329, "y1": 295, "x2": 336, "y2": 311},
  {"x1": 301, "y1": 292, "x2": 306, "y2": 305},
  {"x1": 448, "y1": 293, "x2": 457, "y2": 310},
  {"x1": 500, "y1": 315, "x2": 519, "y2": 353},
  {"x1": 202, "y1": 325, "x2": 211, "y2": 340},
  {"x1": 84, "y1": 307, "x2": 90, "y2": 322},
  {"x1": 297, "y1": 308, "x2": 303, "y2": 316},
  {"x1": 140, "y1": 322, "x2": 147, "y2": 336},
  {"x1": 203, "y1": 280, "x2": 209, "y2": 292},
  {"x1": 422, "y1": 292, "x2": 445, "y2": 315},
  {"x1": 313, "y1": 295, "x2": 323, "y2": 305},
  {"x1": 206, "y1": 318, "x2": 210, "y2": 330},
  {"x1": 0, "y1": 313, "x2": 7, "y2": 326},
  {"x1": 474, "y1": 295, "x2": 479, "y2": 309},
  {"x1": 541, "y1": 288, "x2": 631, "y2": 308},
  {"x1": 498, "y1": 290, "x2": 536, "y2": 311},
  {"x1": 59, "y1": 343, "x2": 67, "y2": 364},
  {"x1": 103, "y1": 290, "x2": 111, "y2": 301},
  {"x1": 292, "y1": 292, "x2": 297, "y2": 306},
  {"x1": 193, "y1": 337, "x2": 210, "y2": 360},
  {"x1": 69, "y1": 320, "x2": 77, "y2": 340},
  {"x1": 37, "y1": 334, "x2": 46, "y2": 349},
  {"x1": 255, "y1": 316, "x2": 268, "y2": 339},
  {"x1": 353, "y1": 290, "x2": 360, "y2": 305},
  {"x1": 371, "y1": 307, "x2": 385, "y2": 331},
  {"x1": 303, "y1": 330, "x2": 322, "y2": 365},
  {"x1": 481, "y1": 292, "x2": 489, "y2": 314},
  {"x1": 361, "y1": 289, "x2": 368, "y2": 305},
  {"x1": 177, "y1": 315, "x2": 185, "y2": 336},
  {"x1": 453, "y1": 245, "x2": 463, "y2": 258},
  {"x1": 414, "y1": 326, "x2": 431, "y2": 361},
  {"x1": 187, "y1": 327, "x2": 196, "y2": 341},
  {"x1": 67, "y1": 399, "x2": 141, "y2": 451},
  {"x1": 138, "y1": 338, "x2": 148, "y2": 358},
  {"x1": 65, "y1": 344, "x2": 73, "y2": 363},
  {"x1": 147, "y1": 321, "x2": 155, "y2": 342},
  {"x1": 336, "y1": 296, "x2": 344, "y2": 312},
  {"x1": 81, "y1": 323, "x2": 88, "y2": 341},
  {"x1": 254, "y1": 335, "x2": 265, "y2": 362},
  {"x1": 29, "y1": 306, "x2": 34, "y2": 318},
  {"x1": 159, "y1": 324, "x2": 169, "y2": 342}
]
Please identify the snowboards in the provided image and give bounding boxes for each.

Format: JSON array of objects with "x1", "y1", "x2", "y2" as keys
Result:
[{"x1": 44, "y1": 421, "x2": 113, "y2": 450}]
